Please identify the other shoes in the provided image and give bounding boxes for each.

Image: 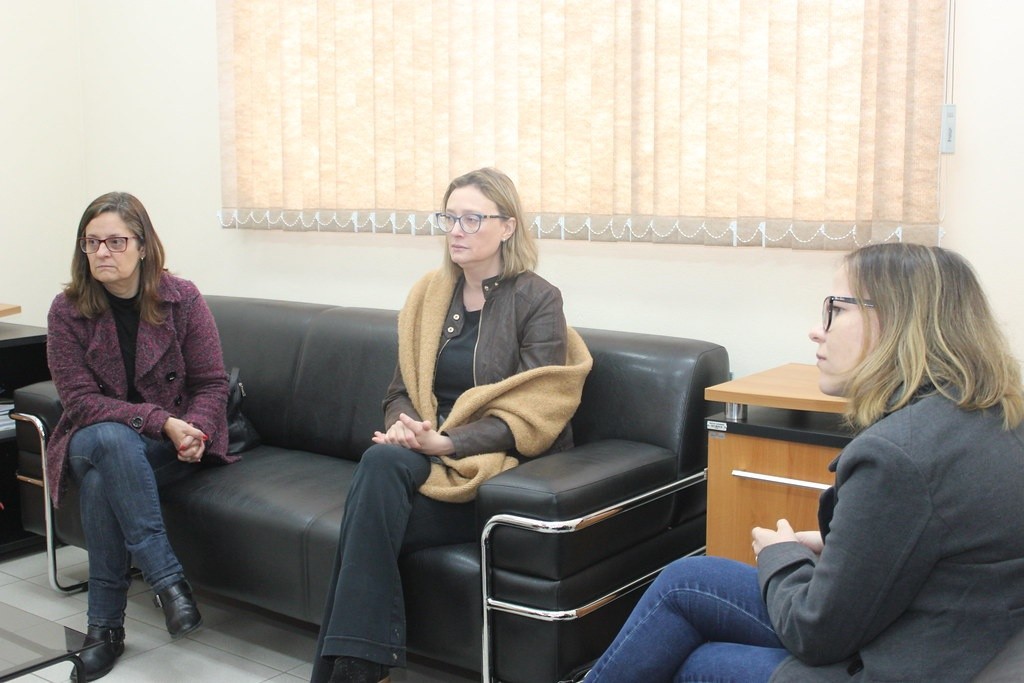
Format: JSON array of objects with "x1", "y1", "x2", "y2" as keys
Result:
[{"x1": 329, "y1": 655, "x2": 391, "y2": 683}]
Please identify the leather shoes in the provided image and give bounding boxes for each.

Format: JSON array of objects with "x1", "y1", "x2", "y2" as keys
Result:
[
  {"x1": 70, "y1": 624, "x2": 125, "y2": 682},
  {"x1": 153, "y1": 579, "x2": 201, "y2": 639}
]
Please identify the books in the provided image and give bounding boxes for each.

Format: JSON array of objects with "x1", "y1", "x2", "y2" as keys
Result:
[{"x1": 0, "y1": 396, "x2": 16, "y2": 431}]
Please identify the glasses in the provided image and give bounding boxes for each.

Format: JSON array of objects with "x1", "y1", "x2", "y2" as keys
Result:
[
  {"x1": 821, "y1": 295, "x2": 875, "y2": 332},
  {"x1": 79, "y1": 236, "x2": 139, "y2": 254},
  {"x1": 435, "y1": 212, "x2": 510, "y2": 234}
]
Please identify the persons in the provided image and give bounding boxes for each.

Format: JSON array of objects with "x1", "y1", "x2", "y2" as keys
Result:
[
  {"x1": 579, "y1": 243, "x2": 1024, "y2": 683},
  {"x1": 311, "y1": 166, "x2": 593, "y2": 683},
  {"x1": 45, "y1": 192, "x2": 242, "y2": 681}
]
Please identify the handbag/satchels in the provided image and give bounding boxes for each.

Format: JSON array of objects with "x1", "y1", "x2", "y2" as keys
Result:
[{"x1": 224, "y1": 367, "x2": 261, "y2": 455}]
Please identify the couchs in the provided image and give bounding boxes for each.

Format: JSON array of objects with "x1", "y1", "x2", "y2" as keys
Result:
[{"x1": 8, "y1": 294, "x2": 731, "y2": 683}]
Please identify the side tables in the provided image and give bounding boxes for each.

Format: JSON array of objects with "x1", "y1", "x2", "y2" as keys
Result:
[
  {"x1": 704, "y1": 364, "x2": 855, "y2": 567},
  {"x1": 0, "y1": 322, "x2": 54, "y2": 555}
]
